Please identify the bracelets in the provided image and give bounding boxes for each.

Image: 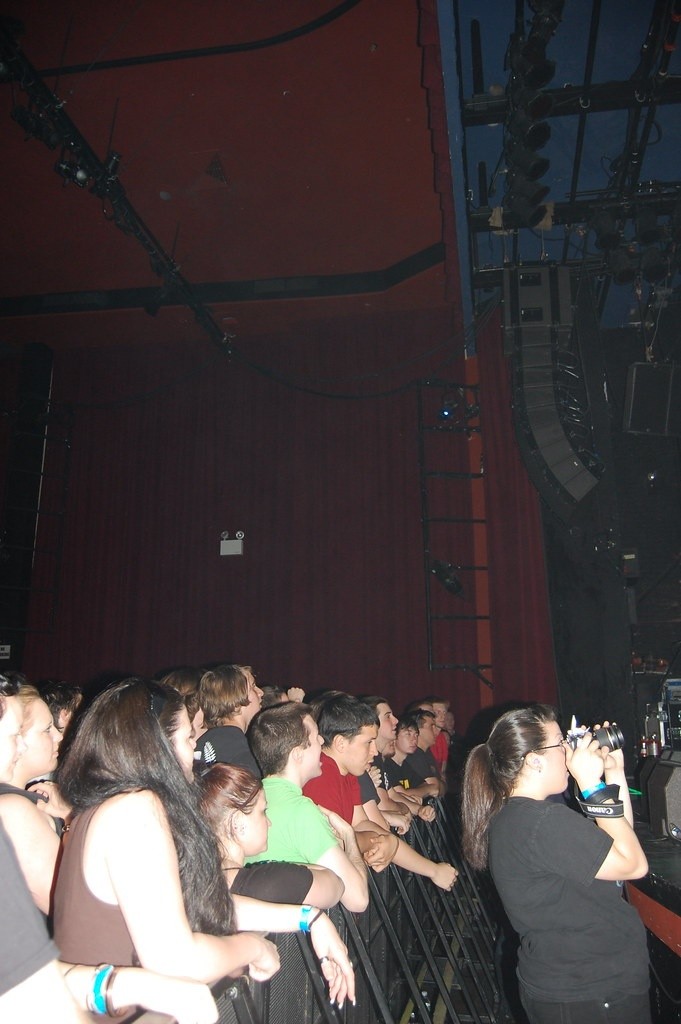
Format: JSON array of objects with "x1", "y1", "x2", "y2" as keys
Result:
[
  {"x1": 582, "y1": 781, "x2": 606, "y2": 800},
  {"x1": 62, "y1": 824, "x2": 70, "y2": 832},
  {"x1": 63, "y1": 964, "x2": 130, "y2": 1019},
  {"x1": 299, "y1": 905, "x2": 324, "y2": 933}
]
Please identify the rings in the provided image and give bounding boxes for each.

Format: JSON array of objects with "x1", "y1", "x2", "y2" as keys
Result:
[{"x1": 318, "y1": 956, "x2": 329, "y2": 964}]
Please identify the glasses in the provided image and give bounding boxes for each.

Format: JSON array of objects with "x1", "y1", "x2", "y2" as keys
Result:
[
  {"x1": 414, "y1": 708, "x2": 424, "y2": 723},
  {"x1": 521, "y1": 740, "x2": 566, "y2": 761}
]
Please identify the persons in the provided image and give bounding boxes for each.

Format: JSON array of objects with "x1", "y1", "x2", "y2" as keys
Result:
[
  {"x1": 460, "y1": 703, "x2": 650, "y2": 1024},
  {"x1": 0, "y1": 664, "x2": 459, "y2": 1024}
]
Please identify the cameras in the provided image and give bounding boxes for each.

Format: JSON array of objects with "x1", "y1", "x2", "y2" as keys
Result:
[{"x1": 568, "y1": 723, "x2": 625, "y2": 754}]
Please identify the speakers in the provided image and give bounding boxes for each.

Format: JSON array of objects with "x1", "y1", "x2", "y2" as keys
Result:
[
  {"x1": 622, "y1": 750, "x2": 681, "y2": 843},
  {"x1": 505, "y1": 264, "x2": 601, "y2": 521},
  {"x1": 623, "y1": 362, "x2": 675, "y2": 437}
]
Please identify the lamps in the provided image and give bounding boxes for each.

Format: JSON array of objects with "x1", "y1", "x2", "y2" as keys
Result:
[
  {"x1": 219, "y1": 530, "x2": 244, "y2": 557},
  {"x1": 506, "y1": 175, "x2": 550, "y2": 204},
  {"x1": 508, "y1": 195, "x2": 547, "y2": 229},
  {"x1": 503, "y1": 112, "x2": 552, "y2": 150},
  {"x1": 437, "y1": 395, "x2": 459, "y2": 418},
  {"x1": 504, "y1": 79, "x2": 554, "y2": 119},
  {"x1": 640, "y1": 246, "x2": 665, "y2": 282},
  {"x1": 502, "y1": 136, "x2": 550, "y2": 182},
  {"x1": 504, "y1": 47, "x2": 555, "y2": 90},
  {"x1": 14, "y1": 106, "x2": 51, "y2": 142},
  {"x1": 431, "y1": 567, "x2": 462, "y2": 593},
  {"x1": 605, "y1": 248, "x2": 637, "y2": 286}
]
